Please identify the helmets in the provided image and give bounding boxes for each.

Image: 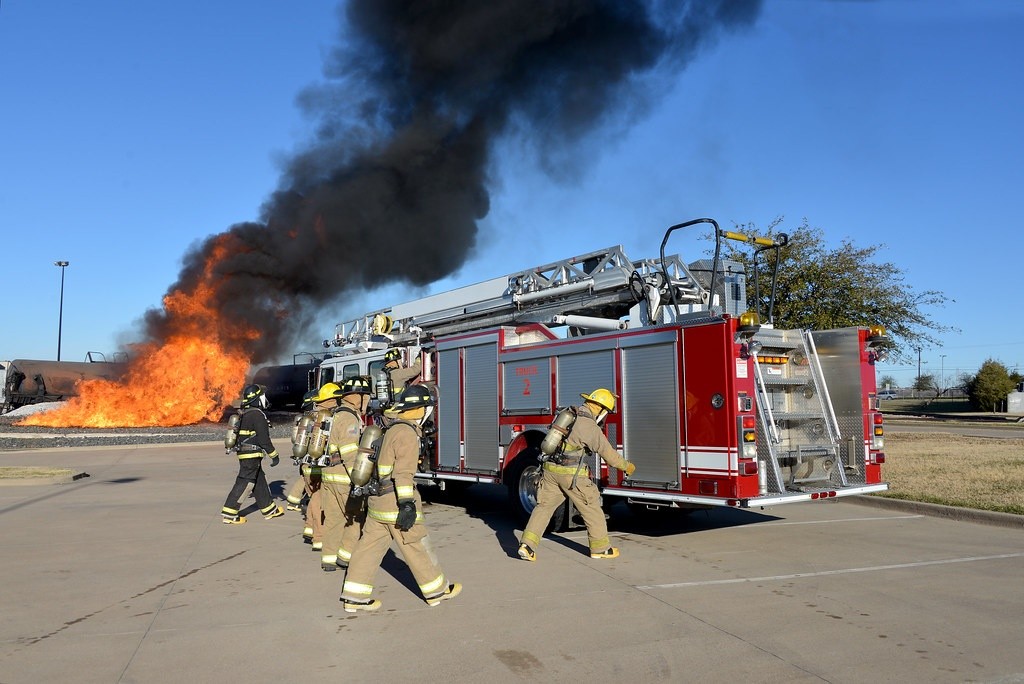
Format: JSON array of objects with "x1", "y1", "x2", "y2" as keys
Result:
[
  {"x1": 580, "y1": 388, "x2": 615, "y2": 413},
  {"x1": 243, "y1": 384, "x2": 267, "y2": 400},
  {"x1": 334, "y1": 376, "x2": 375, "y2": 394},
  {"x1": 301, "y1": 388, "x2": 320, "y2": 411},
  {"x1": 384, "y1": 351, "x2": 399, "y2": 367},
  {"x1": 392, "y1": 385, "x2": 435, "y2": 411}
]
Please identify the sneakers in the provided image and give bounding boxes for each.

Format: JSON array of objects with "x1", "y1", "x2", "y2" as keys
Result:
[
  {"x1": 518, "y1": 544, "x2": 536, "y2": 561},
  {"x1": 427, "y1": 582, "x2": 462, "y2": 606},
  {"x1": 287, "y1": 503, "x2": 301, "y2": 511},
  {"x1": 223, "y1": 516, "x2": 247, "y2": 524},
  {"x1": 265, "y1": 506, "x2": 283, "y2": 519},
  {"x1": 592, "y1": 547, "x2": 619, "y2": 559},
  {"x1": 344, "y1": 598, "x2": 382, "y2": 612}
]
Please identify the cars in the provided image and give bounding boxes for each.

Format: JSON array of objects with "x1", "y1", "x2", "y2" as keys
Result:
[{"x1": 876, "y1": 389, "x2": 898, "y2": 400}]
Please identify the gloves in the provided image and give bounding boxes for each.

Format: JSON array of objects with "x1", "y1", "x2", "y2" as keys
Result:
[
  {"x1": 396, "y1": 501, "x2": 416, "y2": 530},
  {"x1": 270, "y1": 455, "x2": 280, "y2": 466},
  {"x1": 625, "y1": 463, "x2": 635, "y2": 476}
]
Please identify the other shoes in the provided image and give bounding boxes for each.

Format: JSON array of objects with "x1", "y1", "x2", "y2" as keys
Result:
[{"x1": 302, "y1": 536, "x2": 349, "y2": 571}]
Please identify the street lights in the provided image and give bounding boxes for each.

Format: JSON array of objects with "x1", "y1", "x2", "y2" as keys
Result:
[
  {"x1": 54, "y1": 261, "x2": 69, "y2": 361},
  {"x1": 939, "y1": 354, "x2": 947, "y2": 397},
  {"x1": 917, "y1": 346, "x2": 928, "y2": 391}
]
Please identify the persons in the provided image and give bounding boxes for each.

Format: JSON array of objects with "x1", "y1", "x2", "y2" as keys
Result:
[
  {"x1": 322, "y1": 377, "x2": 371, "y2": 570},
  {"x1": 518, "y1": 387, "x2": 635, "y2": 560},
  {"x1": 341, "y1": 385, "x2": 462, "y2": 612},
  {"x1": 220, "y1": 385, "x2": 283, "y2": 524},
  {"x1": 288, "y1": 382, "x2": 342, "y2": 551},
  {"x1": 384, "y1": 347, "x2": 429, "y2": 403}
]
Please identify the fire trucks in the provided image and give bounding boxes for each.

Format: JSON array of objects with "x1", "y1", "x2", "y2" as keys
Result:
[{"x1": 305, "y1": 216, "x2": 892, "y2": 533}]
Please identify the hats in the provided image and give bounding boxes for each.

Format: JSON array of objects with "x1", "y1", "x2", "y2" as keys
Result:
[{"x1": 312, "y1": 383, "x2": 342, "y2": 401}]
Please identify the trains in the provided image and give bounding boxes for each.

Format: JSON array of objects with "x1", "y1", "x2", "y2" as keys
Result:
[{"x1": 0, "y1": 348, "x2": 334, "y2": 416}]
